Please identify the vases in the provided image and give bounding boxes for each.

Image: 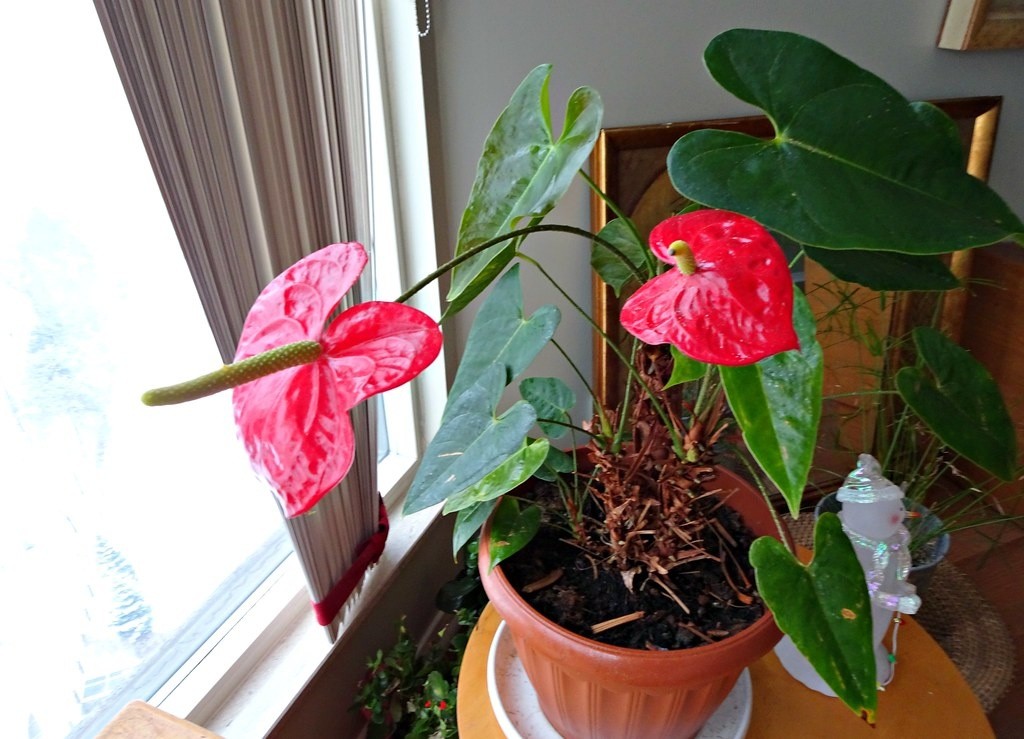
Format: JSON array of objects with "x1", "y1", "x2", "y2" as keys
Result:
[{"x1": 477, "y1": 438, "x2": 798, "y2": 739}]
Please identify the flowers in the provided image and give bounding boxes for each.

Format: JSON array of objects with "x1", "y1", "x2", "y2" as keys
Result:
[{"x1": 138, "y1": 27, "x2": 1024, "y2": 728}]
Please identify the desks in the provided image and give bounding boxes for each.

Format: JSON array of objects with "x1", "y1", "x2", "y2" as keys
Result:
[{"x1": 456, "y1": 542, "x2": 997, "y2": 739}]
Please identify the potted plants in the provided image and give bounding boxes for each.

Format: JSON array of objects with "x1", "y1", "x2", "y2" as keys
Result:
[{"x1": 804, "y1": 275, "x2": 1024, "y2": 596}]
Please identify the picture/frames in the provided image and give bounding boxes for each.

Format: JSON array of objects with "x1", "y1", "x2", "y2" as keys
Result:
[{"x1": 590, "y1": 94, "x2": 1004, "y2": 514}]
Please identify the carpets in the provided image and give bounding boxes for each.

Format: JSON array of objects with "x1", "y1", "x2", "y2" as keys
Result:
[{"x1": 775, "y1": 500, "x2": 1019, "y2": 718}]
